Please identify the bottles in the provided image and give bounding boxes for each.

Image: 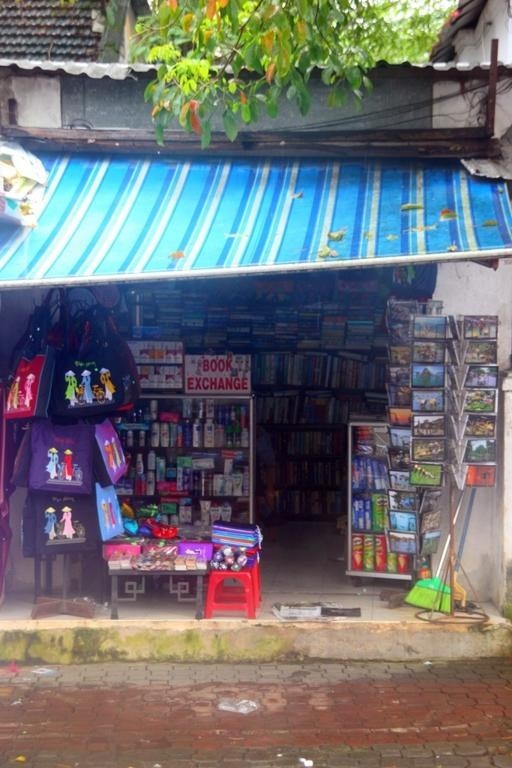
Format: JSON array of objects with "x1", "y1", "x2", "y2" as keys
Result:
[
  {"x1": 177, "y1": 425, "x2": 184, "y2": 447},
  {"x1": 131, "y1": 405, "x2": 150, "y2": 423},
  {"x1": 216, "y1": 402, "x2": 249, "y2": 447},
  {"x1": 135, "y1": 474, "x2": 146, "y2": 495},
  {"x1": 184, "y1": 420, "x2": 192, "y2": 447},
  {"x1": 126, "y1": 429, "x2": 133, "y2": 446},
  {"x1": 169, "y1": 423, "x2": 177, "y2": 447},
  {"x1": 139, "y1": 431, "x2": 146, "y2": 446}
]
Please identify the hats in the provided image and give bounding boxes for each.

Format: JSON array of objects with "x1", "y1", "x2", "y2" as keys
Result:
[
  {"x1": 67, "y1": 287, "x2": 97, "y2": 320},
  {"x1": 92, "y1": 286, "x2": 121, "y2": 309}
]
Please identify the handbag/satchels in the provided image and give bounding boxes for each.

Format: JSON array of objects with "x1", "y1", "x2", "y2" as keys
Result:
[
  {"x1": 95, "y1": 482, "x2": 125, "y2": 542},
  {"x1": 29, "y1": 422, "x2": 96, "y2": 494},
  {"x1": 33, "y1": 495, "x2": 100, "y2": 558},
  {"x1": 9, "y1": 424, "x2": 31, "y2": 487},
  {"x1": 47, "y1": 340, "x2": 141, "y2": 422},
  {"x1": 3, "y1": 353, "x2": 58, "y2": 422},
  {"x1": 8, "y1": 307, "x2": 44, "y2": 380},
  {"x1": 23, "y1": 496, "x2": 32, "y2": 558},
  {"x1": 94, "y1": 419, "x2": 128, "y2": 488},
  {"x1": 47, "y1": 330, "x2": 80, "y2": 356}
]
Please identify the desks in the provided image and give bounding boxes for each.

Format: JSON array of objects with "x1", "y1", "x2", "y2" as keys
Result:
[{"x1": 106, "y1": 570, "x2": 205, "y2": 619}]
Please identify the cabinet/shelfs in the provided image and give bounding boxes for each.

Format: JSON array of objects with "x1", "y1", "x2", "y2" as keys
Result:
[
  {"x1": 111, "y1": 396, "x2": 253, "y2": 524},
  {"x1": 113, "y1": 282, "x2": 387, "y2": 520},
  {"x1": 345, "y1": 421, "x2": 423, "y2": 580}
]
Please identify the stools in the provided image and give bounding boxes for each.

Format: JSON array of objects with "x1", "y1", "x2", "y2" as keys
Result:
[
  {"x1": 203, "y1": 569, "x2": 254, "y2": 618},
  {"x1": 242, "y1": 559, "x2": 261, "y2": 608}
]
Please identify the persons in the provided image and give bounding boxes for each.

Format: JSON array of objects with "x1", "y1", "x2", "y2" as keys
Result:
[
  {"x1": 63, "y1": 370, "x2": 78, "y2": 407},
  {"x1": 42, "y1": 506, "x2": 58, "y2": 540},
  {"x1": 7, "y1": 376, "x2": 22, "y2": 411},
  {"x1": 63, "y1": 448, "x2": 74, "y2": 481},
  {"x1": 103, "y1": 439, "x2": 117, "y2": 472},
  {"x1": 111, "y1": 437, "x2": 123, "y2": 466},
  {"x1": 59, "y1": 506, "x2": 76, "y2": 539},
  {"x1": 46, "y1": 447, "x2": 60, "y2": 480},
  {"x1": 79, "y1": 369, "x2": 93, "y2": 404},
  {"x1": 100, "y1": 367, "x2": 116, "y2": 400},
  {"x1": 99, "y1": 499, "x2": 110, "y2": 527},
  {"x1": 108, "y1": 496, "x2": 117, "y2": 527},
  {"x1": 24, "y1": 372, "x2": 35, "y2": 408}
]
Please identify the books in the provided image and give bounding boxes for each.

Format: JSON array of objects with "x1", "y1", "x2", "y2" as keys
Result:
[
  {"x1": 256, "y1": 459, "x2": 346, "y2": 488},
  {"x1": 345, "y1": 289, "x2": 372, "y2": 353},
  {"x1": 230, "y1": 299, "x2": 252, "y2": 352},
  {"x1": 253, "y1": 353, "x2": 384, "y2": 387},
  {"x1": 211, "y1": 520, "x2": 262, "y2": 551},
  {"x1": 255, "y1": 390, "x2": 388, "y2": 424},
  {"x1": 181, "y1": 290, "x2": 206, "y2": 347},
  {"x1": 252, "y1": 294, "x2": 275, "y2": 349},
  {"x1": 155, "y1": 288, "x2": 180, "y2": 344},
  {"x1": 255, "y1": 429, "x2": 348, "y2": 457},
  {"x1": 120, "y1": 289, "x2": 155, "y2": 339},
  {"x1": 320, "y1": 302, "x2": 346, "y2": 351},
  {"x1": 275, "y1": 298, "x2": 298, "y2": 350},
  {"x1": 204, "y1": 303, "x2": 229, "y2": 347},
  {"x1": 296, "y1": 300, "x2": 320, "y2": 350},
  {"x1": 373, "y1": 301, "x2": 392, "y2": 352},
  {"x1": 257, "y1": 490, "x2": 347, "y2": 517}
]
